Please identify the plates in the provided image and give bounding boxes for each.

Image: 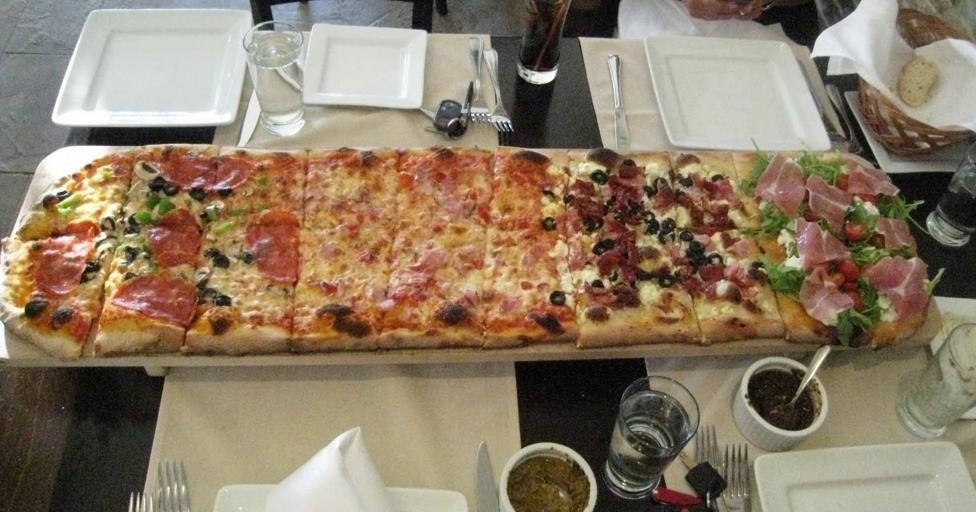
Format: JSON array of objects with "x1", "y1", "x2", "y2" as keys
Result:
[
  {"x1": 754, "y1": 442, "x2": 973, "y2": 510},
  {"x1": 212, "y1": 482, "x2": 468, "y2": 512},
  {"x1": 644, "y1": 34, "x2": 812, "y2": 151},
  {"x1": 50, "y1": 7, "x2": 251, "y2": 126},
  {"x1": 303, "y1": 21, "x2": 428, "y2": 112},
  {"x1": 845, "y1": 87, "x2": 975, "y2": 176}
]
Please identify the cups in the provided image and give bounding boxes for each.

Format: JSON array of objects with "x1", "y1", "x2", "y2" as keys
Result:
[
  {"x1": 896, "y1": 324, "x2": 975, "y2": 439},
  {"x1": 928, "y1": 142, "x2": 976, "y2": 249},
  {"x1": 244, "y1": 19, "x2": 303, "y2": 135},
  {"x1": 605, "y1": 374, "x2": 700, "y2": 502},
  {"x1": 500, "y1": 440, "x2": 599, "y2": 512},
  {"x1": 517, "y1": 0, "x2": 571, "y2": 86}
]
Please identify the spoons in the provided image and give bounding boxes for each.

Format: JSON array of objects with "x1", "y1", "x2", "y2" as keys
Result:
[{"x1": 824, "y1": 84, "x2": 865, "y2": 159}]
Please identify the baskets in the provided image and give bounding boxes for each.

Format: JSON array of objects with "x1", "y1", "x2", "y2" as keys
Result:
[{"x1": 858, "y1": 9, "x2": 976, "y2": 157}]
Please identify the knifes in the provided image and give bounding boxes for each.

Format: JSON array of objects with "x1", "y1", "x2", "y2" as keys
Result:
[
  {"x1": 472, "y1": 442, "x2": 498, "y2": 511},
  {"x1": 606, "y1": 53, "x2": 633, "y2": 155}
]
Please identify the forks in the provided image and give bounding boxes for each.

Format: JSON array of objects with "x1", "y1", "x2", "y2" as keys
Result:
[
  {"x1": 469, "y1": 35, "x2": 514, "y2": 134},
  {"x1": 128, "y1": 458, "x2": 193, "y2": 512},
  {"x1": 686, "y1": 423, "x2": 755, "y2": 512}
]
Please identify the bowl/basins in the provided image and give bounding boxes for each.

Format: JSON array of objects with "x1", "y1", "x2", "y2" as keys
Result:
[{"x1": 733, "y1": 353, "x2": 827, "y2": 448}]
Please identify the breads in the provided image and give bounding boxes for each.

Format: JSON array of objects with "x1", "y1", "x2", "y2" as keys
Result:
[
  {"x1": 897, "y1": 0, "x2": 975, "y2": 49},
  {"x1": 900, "y1": 55, "x2": 940, "y2": 106}
]
